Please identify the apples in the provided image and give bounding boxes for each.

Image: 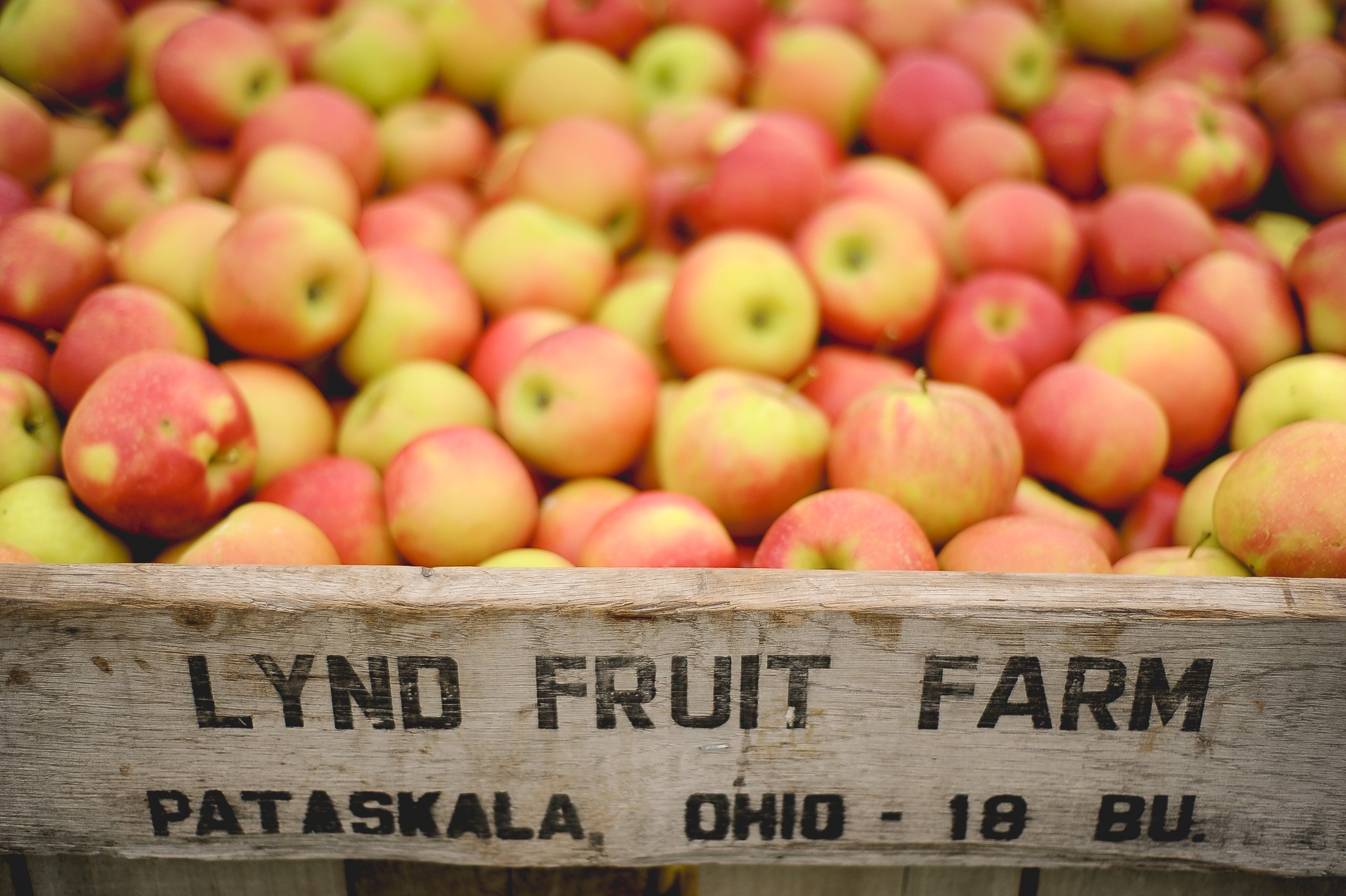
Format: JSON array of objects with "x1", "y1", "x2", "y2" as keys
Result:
[{"x1": 0, "y1": 0, "x2": 1346, "y2": 592}]
